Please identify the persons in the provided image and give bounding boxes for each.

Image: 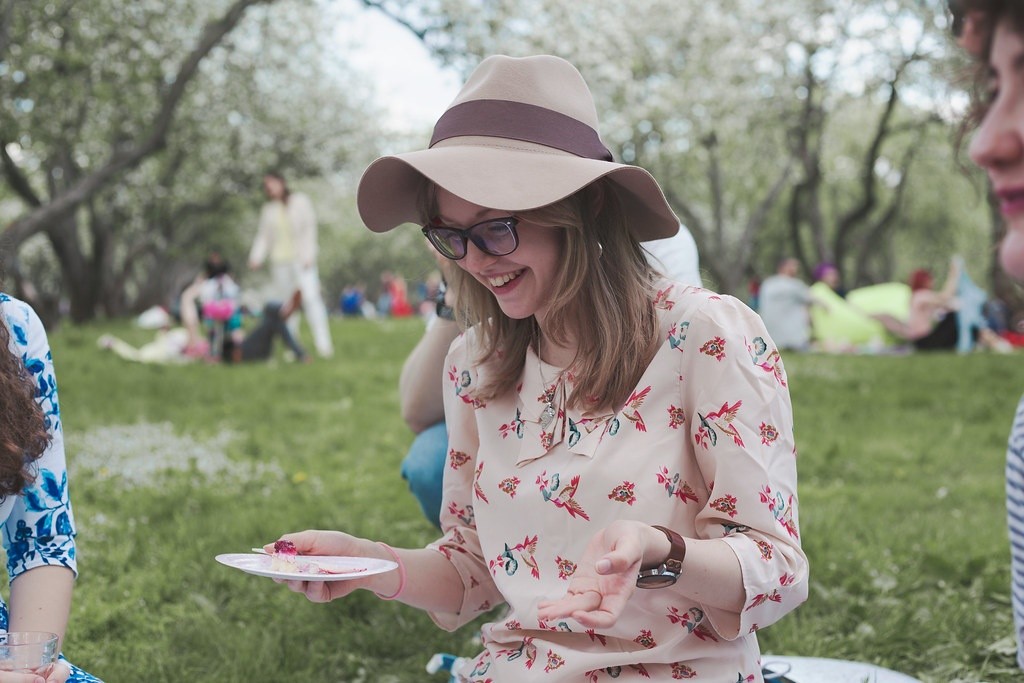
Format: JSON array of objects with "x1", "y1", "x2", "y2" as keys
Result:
[
  {"x1": 0, "y1": 293, "x2": 103, "y2": 683},
  {"x1": 951, "y1": 1, "x2": 1024, "y2": 669},
  {"x1": 249, "y1": 56, "x2": 812, "y2": 683},
  {"x1": 340, "y1": 267, "x2": 446, "y2": 318},
  {"x1": 99, "y1": 169, "x2": 338, "y2": 365},
  {"x1": 758, "y1": 254, "x2": 1005, "y2": 355}
]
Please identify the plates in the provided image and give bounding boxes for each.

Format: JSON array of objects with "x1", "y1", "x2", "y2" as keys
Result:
[{"x1": 215, "y1": 553, "x2": 400, "y2": 581}]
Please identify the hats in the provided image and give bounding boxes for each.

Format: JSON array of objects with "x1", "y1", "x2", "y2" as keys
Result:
[{"x1": 357, "y1": 54, "x2": 680, "y2": 243}]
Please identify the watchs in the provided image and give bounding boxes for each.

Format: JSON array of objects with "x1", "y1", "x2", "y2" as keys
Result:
[{"x1": 636, "y1": 524, "x2": 686, "y2": 589}]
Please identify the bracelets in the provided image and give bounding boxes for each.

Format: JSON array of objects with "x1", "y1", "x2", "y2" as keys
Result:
[
  {"x1": 374, "y1": 541, "x2": 405, "y2": 600},
  {"x1": 436, "y1": 292, "x2": 465, "y2": 323}
]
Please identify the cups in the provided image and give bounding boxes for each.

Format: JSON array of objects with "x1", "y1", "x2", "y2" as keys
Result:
[{"x1": 0, "y1": 631, "x2": 59, "y2": 676}]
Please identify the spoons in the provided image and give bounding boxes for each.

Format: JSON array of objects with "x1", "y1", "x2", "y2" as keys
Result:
[{"x1": 251, "y1": 546, "x2": 367, "y2": 573}]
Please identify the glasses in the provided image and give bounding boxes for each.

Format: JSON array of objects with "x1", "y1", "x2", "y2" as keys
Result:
[{"x1": 422, "y1": 216, "x2": 520, "y2": 260}]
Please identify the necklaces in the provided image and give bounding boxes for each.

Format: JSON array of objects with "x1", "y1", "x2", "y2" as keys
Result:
[{"x1": 538, "y1": 326, "x2": 581, "y2": 417}]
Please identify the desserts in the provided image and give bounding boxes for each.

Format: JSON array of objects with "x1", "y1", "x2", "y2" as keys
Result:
[{"x1": 270, "y1": 540, "x2": 301, "y2": 573}]
